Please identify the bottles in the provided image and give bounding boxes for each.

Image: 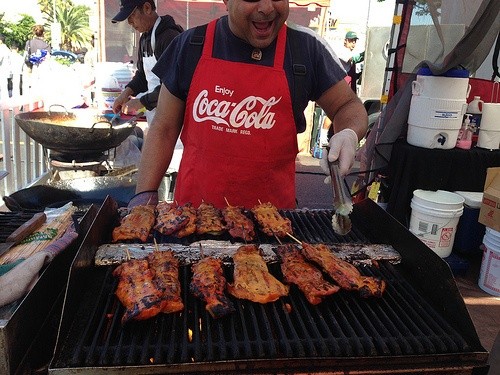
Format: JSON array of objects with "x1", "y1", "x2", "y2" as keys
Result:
[{"x1": 462, "y1": 96, "x2": 484, "y2": 148}]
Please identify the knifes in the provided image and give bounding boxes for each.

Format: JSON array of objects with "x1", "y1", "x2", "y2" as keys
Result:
[{"x1": 0, "y1": 212, "x2": 47, "y2": 256}]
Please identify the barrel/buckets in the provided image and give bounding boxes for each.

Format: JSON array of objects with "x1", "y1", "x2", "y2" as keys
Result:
[
  {"x1": 406, "y1": 66, "x2": 470, "y2": 150},
  {"x1": 478, "y1": 226, "x2": 500, "y2": 298},
  {"x1": 408, "y1": 190, "x2": 465, "y2": 258}
]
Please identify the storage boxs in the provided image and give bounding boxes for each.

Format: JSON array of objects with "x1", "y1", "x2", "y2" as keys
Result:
[{"x1": 479, "y1": 167, "x2": 500, "y2": 232}]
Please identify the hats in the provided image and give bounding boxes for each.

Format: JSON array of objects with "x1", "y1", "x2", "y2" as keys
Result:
[
  {"x1": 111, "y1": 0, "x2": 153, "y2": 23},
  {"x1": 345, "y1": 32, "x2": 359, "y2": 39}
]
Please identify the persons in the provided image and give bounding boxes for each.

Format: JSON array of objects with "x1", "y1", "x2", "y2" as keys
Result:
[
  {"x1": 25, "y1": 24, "x2": 51, "y2": 57},
  {"x1": 111, "y1": 0, "x2": 184, "y2": 129},
  {"x1": 320, "y1": 32, "x2": 365, "y2": 145},
  {"x1": 128, "y1": 0, "x2": 369, "y2": 213},
  {"x1": 0, "y1": 34, "x2": 25, "y2": 96}
]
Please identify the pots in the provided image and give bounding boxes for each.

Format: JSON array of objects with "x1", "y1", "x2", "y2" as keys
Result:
[
  {"x1": 2, "y1": 184, "x2": 81, "y2": 213},
  {"x1": 14, "y1": 104, "x2": 137, "y2": 150}
]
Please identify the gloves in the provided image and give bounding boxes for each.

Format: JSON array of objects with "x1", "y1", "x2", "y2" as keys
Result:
[{"x1": 319, "y1": 128, "x2": 358, "y2": 184}]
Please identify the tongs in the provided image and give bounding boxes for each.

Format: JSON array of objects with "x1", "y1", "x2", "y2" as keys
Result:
[{"x1": 325, "y1": 147, "x2": 354, "y2": 236}]
